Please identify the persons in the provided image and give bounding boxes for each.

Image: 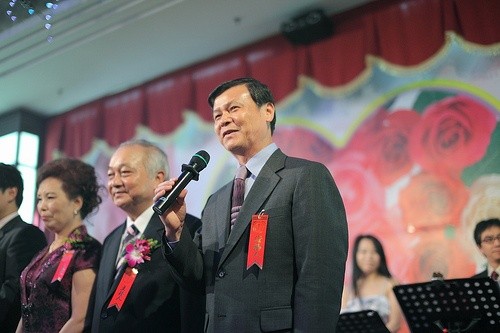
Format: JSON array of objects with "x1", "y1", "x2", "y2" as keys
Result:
[
  {"x1": 0, "y1": 162, "x2": 48, "y2": 333},
  {"x1": 16, "y1": 157, "x2": 109, "y2": 333},
  {"x1": 90, "y1": 139, "x2": 202, "y2": 333},
  {"x1": 341, "y1": 234, "x2": 402, "y2": 333},
  {"x1": 469, "y1": 217, "x2": 500, "y2": 285},
  {"x1": 152, "y1": 75, "x2": 349, "y2": 333}
]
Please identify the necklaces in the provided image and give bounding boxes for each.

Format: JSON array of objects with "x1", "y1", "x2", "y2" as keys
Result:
[{"x1": 49, "y1": 243, "x2": 53, "y2": 254}]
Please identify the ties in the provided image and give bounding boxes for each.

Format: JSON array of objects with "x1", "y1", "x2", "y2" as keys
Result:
[
  {"x1": 113, "y1": 224, "x2": 140, "y2": 283},
  {"x1": 230, "y1": 166, "x2": 250, "y2": 231},
  {"x1": 491, "y1": 272, "x2": 498, "y2": 281}
]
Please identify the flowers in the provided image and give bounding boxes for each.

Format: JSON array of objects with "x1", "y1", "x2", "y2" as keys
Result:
[
  {"x1": 121, "y1": 235, "x2": 158, "y2": 268},
  {"x1": 62, "y1": 229, "x2": 93, "y2": 252}
]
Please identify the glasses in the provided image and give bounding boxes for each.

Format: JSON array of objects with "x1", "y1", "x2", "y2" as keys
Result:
[{"x1": 481, "y1": 233, "x2": 500, "y2": 245}]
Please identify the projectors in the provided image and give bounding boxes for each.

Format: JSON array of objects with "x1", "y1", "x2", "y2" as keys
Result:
[{"x1": 280, "y1": 10, "x2": 335, "y2": 45}]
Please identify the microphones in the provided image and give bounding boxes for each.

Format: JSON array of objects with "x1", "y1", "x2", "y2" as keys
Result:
[{"x1": 152, "y1": 150, "x2": 210, "y2": 216}]
